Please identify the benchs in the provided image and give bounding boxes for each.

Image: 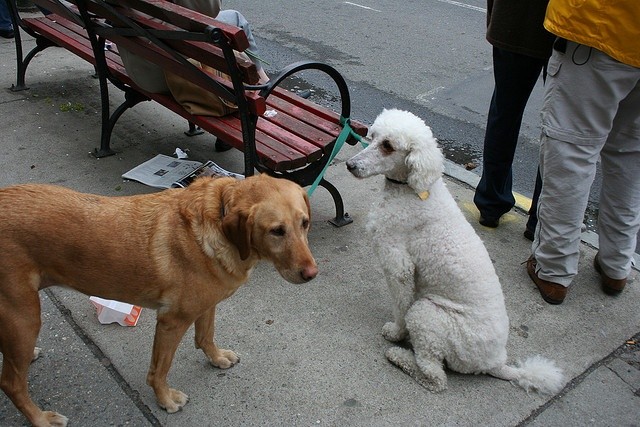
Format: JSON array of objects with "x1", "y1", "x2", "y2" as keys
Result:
[{"x1": 6, "y1": 0, "x2": 368, "y2": 228}]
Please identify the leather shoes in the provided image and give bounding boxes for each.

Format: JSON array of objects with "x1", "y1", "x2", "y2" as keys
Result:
[
  {"x1": 216, "y1": 138, "x2": 232, "y2": 151},
  {"x1": 296, "y1": 89, "x2": 310, "y2": 98}
]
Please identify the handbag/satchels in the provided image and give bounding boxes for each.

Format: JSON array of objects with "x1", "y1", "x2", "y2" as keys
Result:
[{"x1": 164, "y1": 50, "x2": 258, "y2": 116}]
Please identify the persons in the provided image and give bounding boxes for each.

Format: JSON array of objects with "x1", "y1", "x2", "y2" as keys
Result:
[
  {"x1": 106, "y1": 0, "x2": 311, "y2": 152},
  {"x1": 0, "y1": 0, "x2": 15, "y2": 38},
  {"x1": 526, "y1": 0, "x2": 640, "y2": 306},
  {"x1": 473, "y1": 0, "x2": 549, "y2": 242}
]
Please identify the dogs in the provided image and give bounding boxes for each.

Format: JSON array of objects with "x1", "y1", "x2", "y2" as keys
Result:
[
  {"x1": 0, "y1": 172, "x2": 322, "y2": 427},
  {"x1": 343, "y1": 106, "x2": 568, "y2": 398}
]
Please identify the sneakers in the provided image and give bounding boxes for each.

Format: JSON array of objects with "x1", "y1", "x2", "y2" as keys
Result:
[
  {"x1": 0, "y1": 29, "x2": 15, "y2": 38},
  {"x1": 524, "y1": 227, "x2": 534, "y2": 240},
  {"x1": 479, "y1": 214, "x2": 499, "y2": 226},
  {"x1": 527, "y1": 254, "x2": 567, "y2": 304},
  {"x1": 594, "y1": 250, "x2": 626, "y2": 294}
]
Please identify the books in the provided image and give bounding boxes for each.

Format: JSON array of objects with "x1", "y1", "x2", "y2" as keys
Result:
[{"x1": 121, "y1": 153, "x2": 245, "y2": 189}]
